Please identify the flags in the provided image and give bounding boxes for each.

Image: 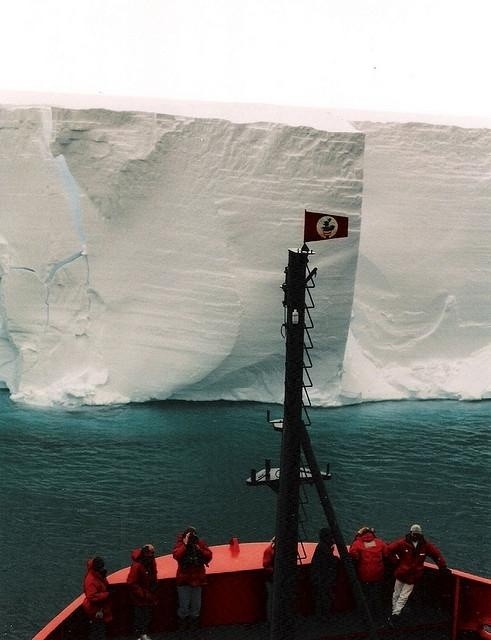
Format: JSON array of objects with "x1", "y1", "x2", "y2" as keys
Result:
[{"x1": 303, "y1": 211, "x2": 349, "y2": 243}]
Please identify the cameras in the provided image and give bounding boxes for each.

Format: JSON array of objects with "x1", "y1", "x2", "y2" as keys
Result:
[{"x1": 189, "y1": 535, "x2": 199, "y2": 544}]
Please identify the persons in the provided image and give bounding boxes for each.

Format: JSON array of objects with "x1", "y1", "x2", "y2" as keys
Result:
[
  {"x1": 306, "y1": 527, "x2": 341, "y2": 624},
  {"x1": 387, "y1": 523, "x2": 453, "y2": 629},
  {"x1": 125, "y1": 543, "x2": 160, "y2": 640},
  {"x1": 348, "y1": 525, "x2": 393, "y2": 628},
  {"x1": 172, "y1": 525, "x2": 214, "y2": 640},
  {"x1": 262, "y1": 534, "x2": 281, "y2": 629},
  {"x1": 81, "y1": 555, "x2": 115, "y2": 640}
]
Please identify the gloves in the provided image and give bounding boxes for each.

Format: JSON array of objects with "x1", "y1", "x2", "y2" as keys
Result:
[{"x1": 439, "y1": 566, "x2": 453, "y2": 579}]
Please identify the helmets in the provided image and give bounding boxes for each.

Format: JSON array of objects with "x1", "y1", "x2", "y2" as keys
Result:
[{"x1": 410, "y1": 524, "x2": 423, "y2": 535}]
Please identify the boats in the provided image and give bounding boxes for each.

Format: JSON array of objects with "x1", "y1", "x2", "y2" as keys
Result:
[{"x1": 30, "y1": 207, "x2": 491, "y2": 640}]
